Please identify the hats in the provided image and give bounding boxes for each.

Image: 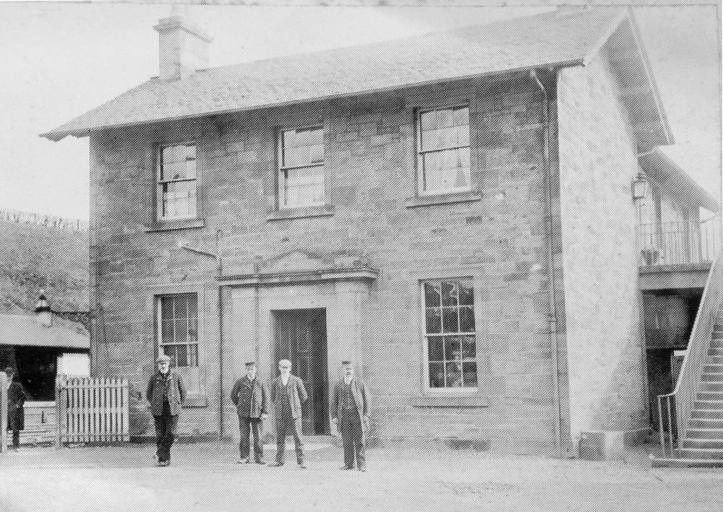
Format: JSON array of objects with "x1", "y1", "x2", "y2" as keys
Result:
[
  {"x1": 155, "y1": 355, "x2": 170, "y2": 362},
  {"x1": 279, "y1": 359, "x2": 291, "y2": 367}
]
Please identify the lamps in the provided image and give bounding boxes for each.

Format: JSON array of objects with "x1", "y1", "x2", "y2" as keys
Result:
[
  {"x1": 35, "y1": 289, "x2": 91, "y2": 330},
  {"x1": 634, "y1": 174, "x2": 647, "y2": 199}
]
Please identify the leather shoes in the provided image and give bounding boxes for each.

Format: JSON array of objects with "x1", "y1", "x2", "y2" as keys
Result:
[
  {"x1": 152, "y1": 454, "x2": 170, "y2": 467},
  {"x1": 359, "y1": 467, "x2": 367, "y2": 472},
  {"x1": 238, "y1": 458, "x2": 283, "y2": 467},
  {"x1": 339, "y1": 465, "x2": 354, "y2": 470},
  {"x1": 299, "y1": 463, "x2": 306, "y2": 467}
]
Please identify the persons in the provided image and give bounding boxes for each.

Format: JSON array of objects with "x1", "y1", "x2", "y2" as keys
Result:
[
  {"x1": 4, "y1": 366, "x2": 27, "y2": 450},
  {"x1": 232, "y1": 361, "x2": 269, "y2": 464},
  {"x1": 266, "y1": 359, "x2": 308, "y2": 468},
  {"x1": 330, "y1": 361, "x2": 370, "y2": 472},
  {"x1": 145, "y1": 355, "x2": 186, "y2": 467}
]
